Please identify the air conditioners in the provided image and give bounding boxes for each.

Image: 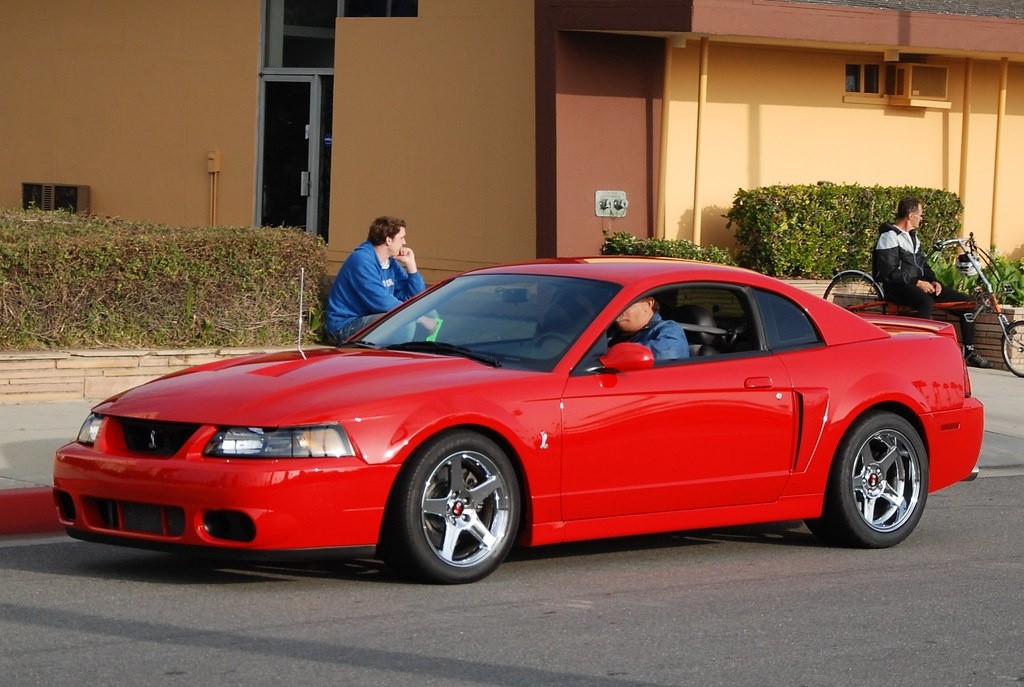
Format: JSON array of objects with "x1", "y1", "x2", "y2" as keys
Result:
[{"x1": 894, "y1": 62, "x2": 950, "y2": 101}]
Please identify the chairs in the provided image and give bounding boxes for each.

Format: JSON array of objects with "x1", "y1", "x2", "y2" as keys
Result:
[
  {"x1": 665, "y1": 305, "x2": 721, "y2": 357},
  {"x1": 534, "y1": 287, "x2": 594, "y2": 356}
]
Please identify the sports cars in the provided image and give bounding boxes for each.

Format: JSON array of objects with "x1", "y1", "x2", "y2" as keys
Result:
[{"x1": 52, "y1": 255, "x2": 985, "y2": 583}]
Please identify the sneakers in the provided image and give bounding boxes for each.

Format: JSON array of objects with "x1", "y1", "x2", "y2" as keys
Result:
[{"x1": 965, "y1": 352, "x2": 994, "y2": 368}]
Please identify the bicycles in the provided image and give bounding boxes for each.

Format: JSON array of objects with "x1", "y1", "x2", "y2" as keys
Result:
[{"x1": 821, "y1": 232, "x2": 1024, "y2": 382}]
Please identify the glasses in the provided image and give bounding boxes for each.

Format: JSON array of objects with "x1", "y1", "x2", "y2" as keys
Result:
[{"x1": 906, "y1": 211, "x2": 924, "y2": 219}]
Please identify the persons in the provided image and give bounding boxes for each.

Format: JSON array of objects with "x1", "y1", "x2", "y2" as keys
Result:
[
  {"x1": 610, "y1": 282, "x2": 690, "y2": 361},
  {"x1": 327, "y1": 216, "x2": 438, "y2": 346},
  {"x1": 873, "y1": 197, "x2": 993, "y2": 368}
]
